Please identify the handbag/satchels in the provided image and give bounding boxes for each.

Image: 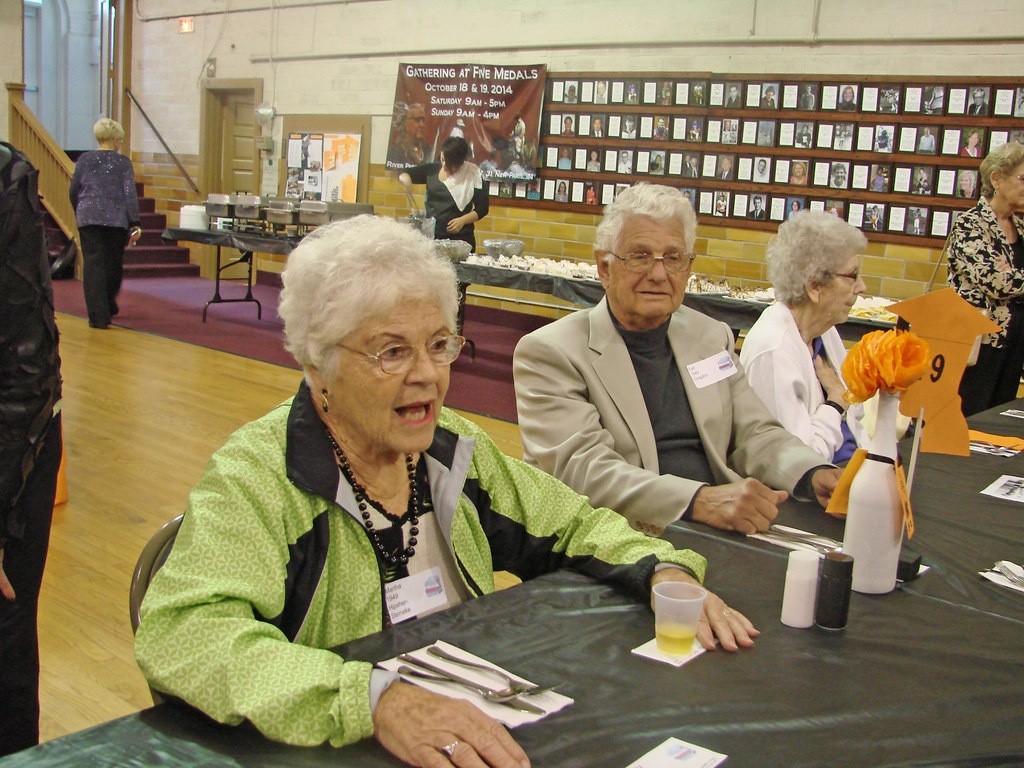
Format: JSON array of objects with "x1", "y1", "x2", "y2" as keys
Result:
[{"x1": 968, "y1": 309, "x2": 987, "y2": 366}]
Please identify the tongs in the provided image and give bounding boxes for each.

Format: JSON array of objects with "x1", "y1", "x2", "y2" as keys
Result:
[{"x1": 405, "y1": 186, "x2": 420, "y2": 217}]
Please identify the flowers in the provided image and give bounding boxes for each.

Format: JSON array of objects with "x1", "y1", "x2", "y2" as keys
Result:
[{"x1": 841, "y1": 326, "x2": 931, "y2": 405}]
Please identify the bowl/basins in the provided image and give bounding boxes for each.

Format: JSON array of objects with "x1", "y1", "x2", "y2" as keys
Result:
[
  {"x1": 483, "y1": 239, "x2": 525, "y2": 260},
  {"x1": 437, "y1": 240, "x2": 472, "y2": 263}
]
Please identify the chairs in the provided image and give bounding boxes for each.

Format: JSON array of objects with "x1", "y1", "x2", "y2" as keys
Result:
[{"x1": 129, "y1": 515, "x2": 181, "y2": 705}]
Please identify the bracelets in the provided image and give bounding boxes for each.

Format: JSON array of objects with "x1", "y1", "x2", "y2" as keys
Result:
[{"x1": 825, "y1": 400, "x2": 848, "y2": 422}]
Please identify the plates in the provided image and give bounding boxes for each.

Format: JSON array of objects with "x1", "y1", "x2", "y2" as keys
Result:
[
  {"x1": 685, "y1": 291, "x2": 732, "y2": 296},
  {"x1": 180, "y1": 204, "x2": 209, "y2": 230},
  {"x1": 848, "y1": 296, "x2": 912, "y2": 329},
  {"x1": 458, "y1": 256, "x2": 602, "y2": 284},
  {"x1": 722, "y1": 295, "x2": 773, "y2": 305}
]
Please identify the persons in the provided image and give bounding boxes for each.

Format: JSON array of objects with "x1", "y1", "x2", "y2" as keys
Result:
[
  {"x1": 562, "y1": 116, "x2": 773, "y2": 145},
  {"x1": 450, "y1": 119, "x2": 465, "y2": 139},
  {"x1": 0, "y1": 139, "x2": 69, "y2": 755},
  {"x1": 512, "y1": 186, "x2": 847, "y2": 542},
  {"x1": 944, "y1": 140, "x2": 1023, "y2": 419},
  {"x1": 790, "y1": 161, "x2": 977, "y2": 198},
  {"x1": 497, "y1": 178, "x2": 629, "y2": 206},
  {"x1": 388, "y1": 104, "x2": 429, "y2": 167},
  {"x1": 70, "y1": 119, "x2": 141, "y2": 330},
  {"x1": 559, "y1": 148, "x2": 772, "y2": 183},
  {"x1": 795, "y1": 124, "x2": 1024, "y2": 157},
  {"x1": 134, "y1": 212, "x2": 760, "y2": 768},
  {"x1": 884, "y1": 88, "x2": 1024, "y2": 116},
  {"x1": 513, "y1": 115, "x2": 525, "y2": 165},
  {"x1": 563, "y1": 80, "x2": 740, "y2": 109},
  {"x1": 685, "y1": 190, "x2": 963, "y2": 238},
  {"x1": 732, "y1": 202, "x2": 873, "y2": 467},
  {"x1": 762, "y1": 85, "x2": 857, "y2": 110},
  {"x1": 395, "y1": 135, "x2": 491, "y2": 337}
]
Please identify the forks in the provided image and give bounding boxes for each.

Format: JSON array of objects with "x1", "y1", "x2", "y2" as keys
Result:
[
  {"x1": 994, "y1": 561, "x2": 1024, "y2": 588},
  {"x1": 969, "y1": 439, "x2": 1020, "y2": 449},
  {"x1": 428, "y1": 645, "x2": 567, "y2": 698},
  {"x1": 770, "y1": 525, "x2": 843, "y2": 548}
]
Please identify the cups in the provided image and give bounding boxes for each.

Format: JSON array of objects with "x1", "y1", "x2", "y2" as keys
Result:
[{"x1": 652, "y1": 581, "x2": 708, "y2": 657}]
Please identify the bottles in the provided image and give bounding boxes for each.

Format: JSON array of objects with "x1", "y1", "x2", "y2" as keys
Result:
[
  {"x1": 780, "y1": 550, "x2": 820, "y2": 628},
  {"x1": 814, "y1": 552, "x2": 854, "y2": 632}
]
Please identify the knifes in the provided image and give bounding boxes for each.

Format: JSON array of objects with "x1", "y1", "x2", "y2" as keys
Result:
[
  {"x1": 969, "y1": 445, "x2": 1020, "y2": 453},
  {"x1": 759, "y1": 528, "x2": 839, "y2": 549},
  {"x1": 399, "y1": 652, "x2": 546, "y2": 716}
]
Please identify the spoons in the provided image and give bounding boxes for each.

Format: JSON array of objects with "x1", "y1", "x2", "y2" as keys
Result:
[
  {"x1": 969, "y1": 443, "x2": 1003, "y2": 453},
  {"x1": 758, "y1": 530, "x2": 836, "y2": 558},
  {"x1": 397, "y1": 665, "x2": 528, "y2": 704}
]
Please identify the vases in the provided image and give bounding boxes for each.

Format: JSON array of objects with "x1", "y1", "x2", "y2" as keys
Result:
[{"x1": 842, "y1": 387, "x2": 904, "y2": 595}]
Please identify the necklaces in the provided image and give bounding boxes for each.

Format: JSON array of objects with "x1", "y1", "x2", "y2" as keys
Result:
[{"x1": 324, "y1": 426, "x2": 422, "y2": 566}]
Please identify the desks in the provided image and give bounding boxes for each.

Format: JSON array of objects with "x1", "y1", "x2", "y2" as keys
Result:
[
  {"x1": 160, "y1": 228, "x2": 904, "y2": 364},
  {"x1": 0, "y1": 529, "x2": 1023, "y2": 768},
  {"x1": 668, "y1": 400, "x2": 1024, "y2": 622}
]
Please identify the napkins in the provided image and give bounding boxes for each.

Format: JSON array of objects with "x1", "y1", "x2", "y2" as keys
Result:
[
  {"x1": 970, "y1": 442, "x2": 1020, "y2": 458},
  {"x1": 378, "y1": 640, "x2": 575, "y2": 729},
  {"x1": 747, "y1": 525, "x2": 931, "y2": 582},
  {"x1": 1000, "y1": 409, "x2": 1024, "y2": 418},
  {"x1": 979, "y1": 560, "x2": 1023, "y2": 592}
]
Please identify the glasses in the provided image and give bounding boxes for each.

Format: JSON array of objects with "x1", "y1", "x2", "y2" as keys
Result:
[
  {"x1": 609, "y1": 245, "x2": 697, "y2": 277},
  {"x1": 830, "y1": 269, "x2": 862, "y2": 282},
  {"x1": 337, "y1": 332, "x2": 467, "y2": 377}
]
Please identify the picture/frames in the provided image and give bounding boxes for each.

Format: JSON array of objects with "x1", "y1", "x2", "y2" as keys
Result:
[{"x1": 497, "y1": 80, "x2": 1024, "y2": 241}]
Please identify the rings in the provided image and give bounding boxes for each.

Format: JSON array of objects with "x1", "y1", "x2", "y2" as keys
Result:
[
  {"x1": 722, "y1": 608, "x2": 732, "y2": 617},
  {"x1": 440, "y1": 741, "x2": 460, "y2": 756}
]
